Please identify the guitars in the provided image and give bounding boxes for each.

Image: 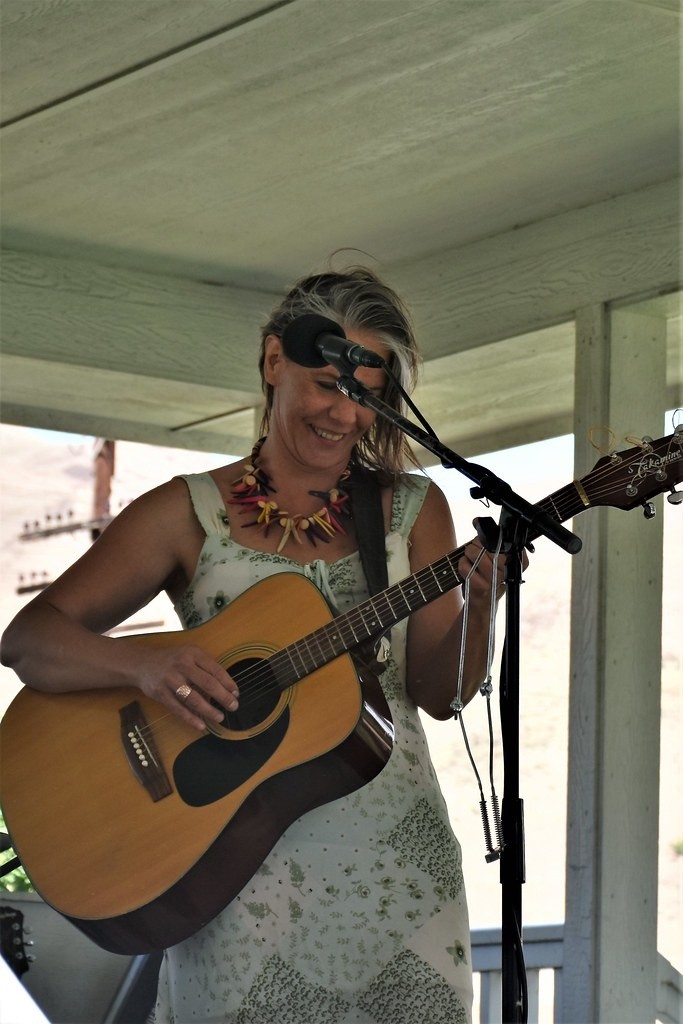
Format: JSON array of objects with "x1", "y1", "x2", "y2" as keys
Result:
[
  {"x1": 0, "y1": 905, "x2": 37, "y2": 981},
  {"x1": 0, "y1": 424, "x2": 682, "y2": 956}
]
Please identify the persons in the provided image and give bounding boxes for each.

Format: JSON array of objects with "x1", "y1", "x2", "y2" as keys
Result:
[{"x1": 0, "y1": 246, "x2": 528, "y2": 1024}]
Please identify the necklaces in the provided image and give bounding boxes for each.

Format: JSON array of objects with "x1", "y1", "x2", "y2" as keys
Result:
[{"x1": 225, "y1": 436, "x2": 352, "y2": 553}]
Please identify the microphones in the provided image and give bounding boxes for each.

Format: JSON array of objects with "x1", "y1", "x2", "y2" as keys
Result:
[{"x1": 282, "y1": 314, "x2": 386, "y2": 368}]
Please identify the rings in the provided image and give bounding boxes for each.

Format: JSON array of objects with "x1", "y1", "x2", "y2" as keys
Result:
[
  {"x1": 499, "y1": 580, "x2": 506, "y2": 589},
  {"x1": 175, "y1": 684, "x2": 192, "y2": 701}
]
